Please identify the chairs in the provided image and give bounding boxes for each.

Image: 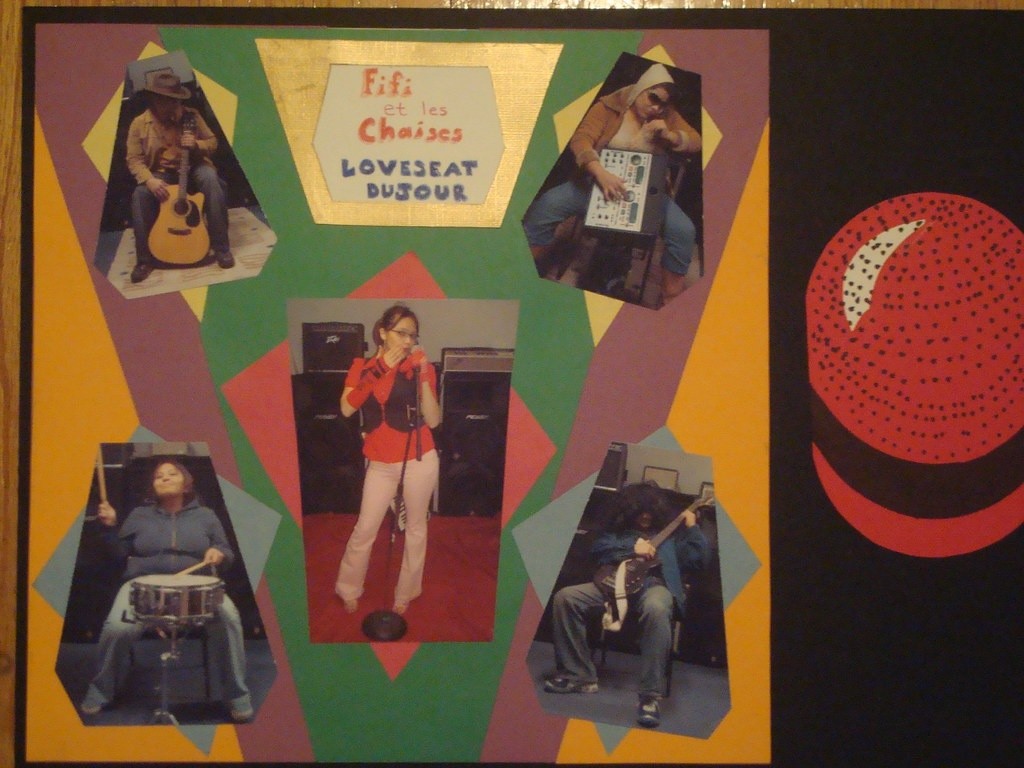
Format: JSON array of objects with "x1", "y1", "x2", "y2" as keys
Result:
[
  {"x1": 590, "y1": 597, "x2": 685, "y2": 698},
  {"x1": 570, "y1": 154, "x2": 687, "y2": 305},
  {"x1": 112, "y1": 627, "x2": 211, "y2": 705}
]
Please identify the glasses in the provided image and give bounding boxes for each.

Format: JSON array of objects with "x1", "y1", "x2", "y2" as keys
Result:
[
  {"x1": 646, "y1": 89, "x2": 667, "y2": 113},
  {"x1": 386, "y1": 329, "x2": 418, "y2": 340}
]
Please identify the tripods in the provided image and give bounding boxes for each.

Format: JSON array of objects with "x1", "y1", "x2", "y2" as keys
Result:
[{"x1": 147, "y1": 626, "x2": 181, "y2": 726}]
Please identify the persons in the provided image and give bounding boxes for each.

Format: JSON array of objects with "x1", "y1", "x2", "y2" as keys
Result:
[
  {"x1": 336, "y1": 306, "x2": 442, "y2": 613},
  {"x1": 77, "y1": 459, "x2": 258, "y2": 723},
  {"x1": 543, "y1": 479, "x2": 709, "y2": 728},
  {"x1": 124, "y1": 71, "x2": 234, "y2": 282},
  {"x1": 527, "y1": 63, "x2": 705, "y2": 306}
]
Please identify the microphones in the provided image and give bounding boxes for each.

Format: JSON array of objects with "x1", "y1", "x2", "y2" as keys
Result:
[{"x1": 411, "y1": 345, "x2": 425, "y2": 374}]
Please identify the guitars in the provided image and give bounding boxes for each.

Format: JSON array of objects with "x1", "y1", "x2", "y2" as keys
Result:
[
  {"x1": 593, "y1": 495, "x2": 714, "y2": 602},
  {"x1": 147, "y1": 111, "x2": 212, "y2": 266}
]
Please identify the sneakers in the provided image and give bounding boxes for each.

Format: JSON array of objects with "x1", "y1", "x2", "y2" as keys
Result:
[
  {"x1": 637, "y1": 695, "x2": 662, "y2": 726},
  {"x1": 546, "y1": 675, "x2": 599, "y2": 694}
]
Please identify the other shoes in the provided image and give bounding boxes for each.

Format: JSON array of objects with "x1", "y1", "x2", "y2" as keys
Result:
[
  {"x1": 232, "y1": 696, "x2": 253, "y2": 723},
  {"x1": 392, "y1": 599, "x2": 409, "y2": 613},
  {"x1": 215, "y1": 246, "x2": 234, "y2": 268},
  {"x1": 345, "y1": 599, "x2": 357, "y2": 613},
  {"x1": 81, "y1": 696, "x2": 102, "y2": 715},
  {"x1": 130, "y1": 258, "x2": 156, "y2": 282}
]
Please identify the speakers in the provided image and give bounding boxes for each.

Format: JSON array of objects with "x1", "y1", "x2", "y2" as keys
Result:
[
  {"x1": 290, "y1": 372, "x2": 367, "y2": 516},
  {"x1": 302, "y1": 322, "x2": 365, "y2": 371},
  {"x1": 439, "y1": 371, "x2": 512, "y2": 517},
  {"x1": 534, "y1": 490, "x2": 616, "y2": 645}
]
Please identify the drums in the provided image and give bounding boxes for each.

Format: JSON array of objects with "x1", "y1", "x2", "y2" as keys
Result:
[{"x1": 131, "y1": 573, "x2": 225, "y2": 623}]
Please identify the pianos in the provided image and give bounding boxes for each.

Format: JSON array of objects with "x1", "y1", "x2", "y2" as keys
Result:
[{"x1": 389, "y1": 496, "x2": 433, "y2": 533}]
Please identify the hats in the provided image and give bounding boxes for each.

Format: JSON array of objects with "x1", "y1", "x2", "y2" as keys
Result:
[{"x1": 144, "y1": 68, "x2": 191, "y2": 99}]
